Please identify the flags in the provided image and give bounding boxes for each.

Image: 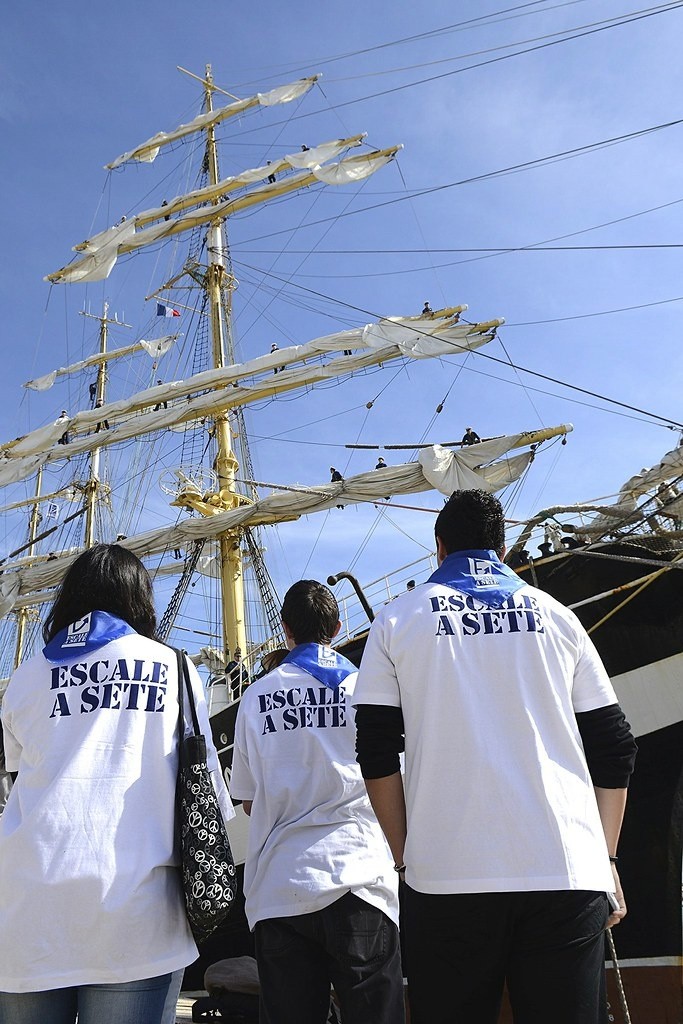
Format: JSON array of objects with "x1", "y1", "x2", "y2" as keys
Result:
[{"x1": 156, "y1": 303, "x2": 180, "y2": 317}]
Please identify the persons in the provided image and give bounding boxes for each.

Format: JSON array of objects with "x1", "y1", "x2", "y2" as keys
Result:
[
  {"x1": 376, "y1": 456, "x2": 391, "y2": 500},
  {"x1": 341, "y1": 330, "x2": 352, "y2": 356},
  {"x1": 162, "y1": 201, "x2": 170, "y2": 221},
  {"x1": 0, "y1": 544, "x2": 236, "y2": 1024},
  {"x1": 174, "y1": 549, "x2": 182, "y2": 559},
  {"x1": 223, "y1": 651, "x2": 250, "y2": 700},
  {"x1": 94, "y1": 398, "x2": 109, "y2": 434},
  {"x1": 422, "y1": 301, "x2": 432, "y2": 314},
  {"x1": 301, "y1": 144, "x2": 310, "y2": 151},
  {"x1": 266, "y1": 160, "x2": 276, "y2": 184},
  {"x1": 59, "y1": 409, "x2": 69, "y2": 445},
  {"x1": 229, "y1": 579, "x2": 406, "y2": 1024},
  {"x1": 461, "y1": 427, "x2": 482, "y2": 469},
  {"x1": 153, "y1": 379, "x2": 167, "y2": 411},
  {"x1": 351, "y1": 489, "x2": 638, "y2": 1024},
  {"x1": 47, "y1": 552, "x2": 57, "y2": 589},
  {"x1": 330, "y1": 466, "x2": 345, "y2": 510},
  {"x1": 271, "y1": 343, "x2": 285, "y2": 374}
]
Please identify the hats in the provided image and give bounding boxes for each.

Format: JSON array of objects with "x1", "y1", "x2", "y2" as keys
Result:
[
  {"x1": 121, "y1": 215, "x2": 126, "y2": 219},
  {"x1": 301, "y1": 144, "x2": 307, "y2": 149},
  {"x1": 156, "y1": 379, "x2": 162, "y2": 383},
  {"x1": 271, "y1": 343, "x2": 277, "y2": 347},
  {"x1": 330, "y1": 466, "x2": 335, "y2": 470},
  {"x1": 378, "y1": 456, "x2": 384, "y2": 461},
  {"x1": 267, "y1": 160, "x2": 272, "y2": 163},
  {"x1": 163, "y1": 199, "x2": 166, "y2": 202},
  {"x1": 62, "y1": 409, "x2": 67, "y2": 413},
  {"x1": 97, "y1": 398, "x2": 102, "y2": 401},
  {"x1": 424, "y1": 302, "x2": 429, "y2": 306},
  {"x1": 465, "y1": 427, "x2": 472, "y2": 431},
  {"x1": 117, "y1": 534, "x2": 124, "y2": 538}
]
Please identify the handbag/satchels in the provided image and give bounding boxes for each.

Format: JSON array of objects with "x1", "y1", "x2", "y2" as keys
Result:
[{"x1": 174, "y1": 650, "x2": 237, "y2": 944}]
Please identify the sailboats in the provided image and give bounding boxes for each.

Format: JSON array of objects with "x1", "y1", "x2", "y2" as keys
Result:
[{"x1": 0, "y1": 62, "x2": 683, "y2": 1024}]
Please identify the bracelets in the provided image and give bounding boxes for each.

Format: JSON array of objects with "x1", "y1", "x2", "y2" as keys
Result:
[
  {"x1": 610, "y1": 856, "x2": 618, "y2": 862},
  {"x1": 394, "y1": 863, "x2": 405, "y2": 872}
]
